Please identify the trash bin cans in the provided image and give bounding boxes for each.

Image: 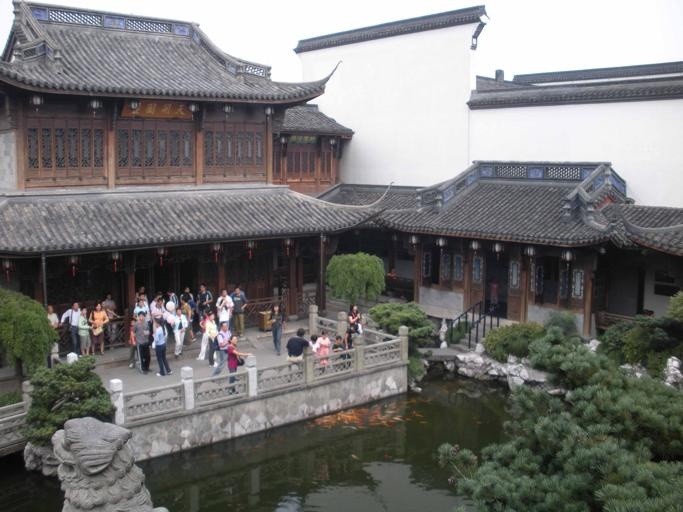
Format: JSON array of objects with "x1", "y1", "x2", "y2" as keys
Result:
[{"x1": 258, "y1": 311, "x2": 273, "y2": 331}]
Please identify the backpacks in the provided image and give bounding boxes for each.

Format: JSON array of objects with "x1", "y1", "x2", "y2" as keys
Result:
[{"x1": 213, "y1": 333, "x2": 225, "y2": 351}]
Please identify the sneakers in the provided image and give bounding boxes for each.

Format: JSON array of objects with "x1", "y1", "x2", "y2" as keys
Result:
[{"x1": 129, "y1": 362, "x2": 175, "y2": 377}]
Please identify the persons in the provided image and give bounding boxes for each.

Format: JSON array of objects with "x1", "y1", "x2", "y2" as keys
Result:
[
  {"x1": 285, "y1": 327, "x2": 308, "y2": 384},
  {"x1": 267, "y1": 301, "x2": 286, "y2": 356},
  {"x1": 487, "y1": 277, "x2": 498, "y2": 312},
  {"x1": 44, "y1": 276, "x2": 251, "y2": 406},
  {"x1": 307, "y1": 303, "x2": 364, "y2": 375}
]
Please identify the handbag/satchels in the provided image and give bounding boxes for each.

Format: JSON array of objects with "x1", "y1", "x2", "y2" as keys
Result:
[
  {"x1": 91, "y1": 323, "x2": 103, "y2": 336},
  {"x1": 237, "y1": 357, "x2": 245, "y2": 366}
]
